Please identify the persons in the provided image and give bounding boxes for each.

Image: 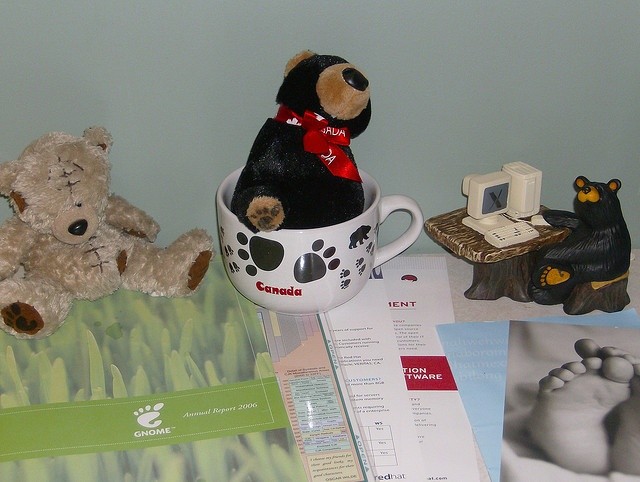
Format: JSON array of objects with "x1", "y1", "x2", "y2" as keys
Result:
[{"x1": 531, "y1": 339, "x2": 640, "y2": 476}]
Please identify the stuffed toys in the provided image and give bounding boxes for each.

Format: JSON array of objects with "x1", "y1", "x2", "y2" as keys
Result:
[
  {"x1": 230, "y1": 49, "x2": 371, "y2": 233},
  {"x1": 0, "y1": 125, "x2": 216, "y2": 340}
]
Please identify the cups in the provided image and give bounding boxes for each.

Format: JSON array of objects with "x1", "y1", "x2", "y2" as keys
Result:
[{"x1": 215, "y1": 166, "x2": 425, "y2": 314}]
